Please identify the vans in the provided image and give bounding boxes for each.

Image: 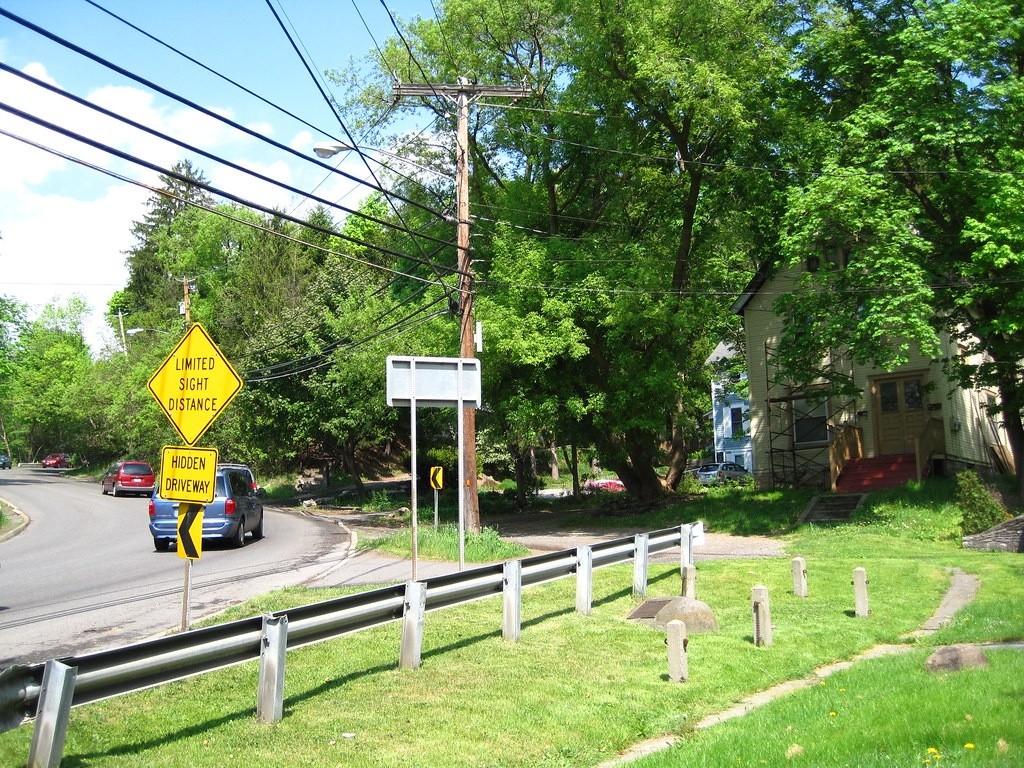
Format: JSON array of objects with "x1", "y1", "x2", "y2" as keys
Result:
[{"x1": 149, "y1": 462, "x2": 267, "y2": 552}]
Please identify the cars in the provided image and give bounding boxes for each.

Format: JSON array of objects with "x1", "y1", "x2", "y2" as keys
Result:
[
  {"x1": 0, "y1": 454, "x2": 12, "y2": 469},
  {"x1": 696, "y1": 461, "x2": 751, "y2": 487},
  {"x1": 102, "y1": 460, "x2": 156, "y2": 499},
  {"x1": 43, "y1": 452, "x2": 73, "y2": 469}
]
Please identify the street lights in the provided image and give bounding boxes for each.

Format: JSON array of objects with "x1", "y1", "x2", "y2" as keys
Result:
[{"x1": 313, "y1": 144, "x2": 481, "y2": 538}]
